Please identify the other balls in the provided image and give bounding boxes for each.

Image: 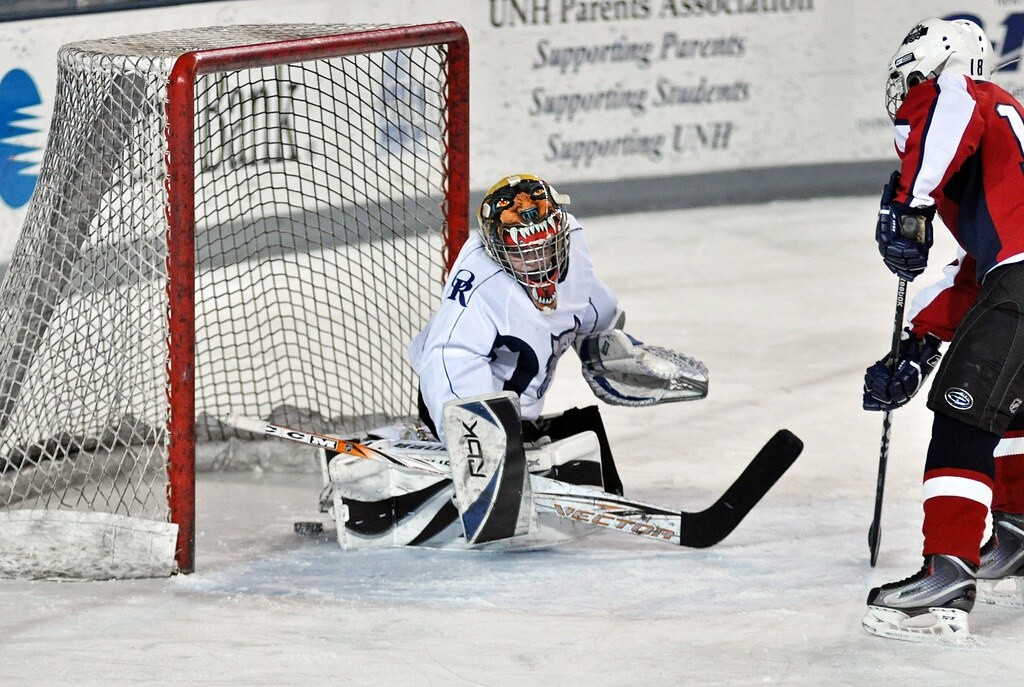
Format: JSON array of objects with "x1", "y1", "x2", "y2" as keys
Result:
[{"x1": 293, "y1": 520, "x2": 323, "y2": 536}]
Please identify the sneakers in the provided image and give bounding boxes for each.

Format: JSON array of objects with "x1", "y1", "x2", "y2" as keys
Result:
[
  {"x1": 975, "y1": 509, "x2": 1024, "y2": 608},
  {"x1": 862, "y1": 552, "x2": 979, "y2": 645}
]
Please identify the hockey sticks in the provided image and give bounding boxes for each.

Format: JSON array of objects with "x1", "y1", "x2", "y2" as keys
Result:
[
  {"x1": 866, "y1": 276, "x2": 909, "y2": 570},
  {"x1": 235, "y1": 415, "x2": 805, "y2": 550}
]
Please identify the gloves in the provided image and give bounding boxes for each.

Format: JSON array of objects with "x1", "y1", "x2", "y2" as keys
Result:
[
  {"x1": 875, "y1": 169, "x2": 936, "y2": 281},
  {"x1": 863, "y1": 326, "x2": 941, "y2": 411}
]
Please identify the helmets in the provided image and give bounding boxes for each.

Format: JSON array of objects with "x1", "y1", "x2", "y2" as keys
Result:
[
  {"x1": 885, "y1": 17, "x2": 992, "y2": 120},
  {"x1": 477, "y1": 174, "x2": 571, "y2": 287}
]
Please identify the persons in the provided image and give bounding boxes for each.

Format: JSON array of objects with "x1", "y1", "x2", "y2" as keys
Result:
[
  {"x1": 862, "y1": 19, "x2": 1024, "y2": 644},
  {"x1": 318, "y1": 174, "x2": 708, "y2": 553}
]
